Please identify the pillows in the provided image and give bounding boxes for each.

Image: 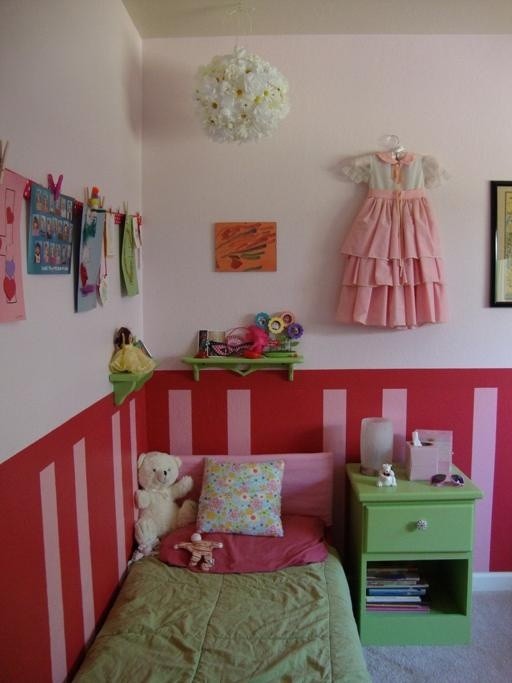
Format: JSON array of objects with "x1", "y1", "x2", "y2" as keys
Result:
[{"x1": 155, "y1": 448, "x2": 337, "y2": 575}]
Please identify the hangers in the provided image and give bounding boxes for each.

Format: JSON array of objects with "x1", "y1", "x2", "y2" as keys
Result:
[{"x1": 383, "y1": 134, "x2": 407, "y2": 157}]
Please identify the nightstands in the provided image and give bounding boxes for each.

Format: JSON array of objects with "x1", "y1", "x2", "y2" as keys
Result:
[{"x1": 346, "y1": 460, "x2": 483, "y2": 647}]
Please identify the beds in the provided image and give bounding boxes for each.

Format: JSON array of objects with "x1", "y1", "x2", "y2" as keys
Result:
[{"x1": 65, "y1": 498, "x2": 374, "y2": 682}]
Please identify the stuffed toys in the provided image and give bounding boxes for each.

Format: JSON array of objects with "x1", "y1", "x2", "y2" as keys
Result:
[
  {"x1": 108, "y1": 327, "x2": 155, "y2": 376},
  {"x1": 171, "y1": 532, "x2": 225, "y2": 572},
  {"x1": 376, "y1": 462, "x2": 399, "y2": 489},
  {"x1": 134, "y1": 451, "x2": 198, "y2": 560}
]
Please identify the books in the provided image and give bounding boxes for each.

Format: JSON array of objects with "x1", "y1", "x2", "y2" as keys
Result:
[{"x1": 366, "y1": 567, "x2": 432, "y2": 613}]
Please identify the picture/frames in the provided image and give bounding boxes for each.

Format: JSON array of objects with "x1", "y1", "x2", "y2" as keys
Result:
[{"x1": 489, "y1": 179, "x2": 512, "y2": 308}]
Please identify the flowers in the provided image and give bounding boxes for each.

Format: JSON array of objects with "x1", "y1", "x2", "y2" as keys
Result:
[{"x1": 193, "y1": 45, "x2": 289, "y2": 146}]
